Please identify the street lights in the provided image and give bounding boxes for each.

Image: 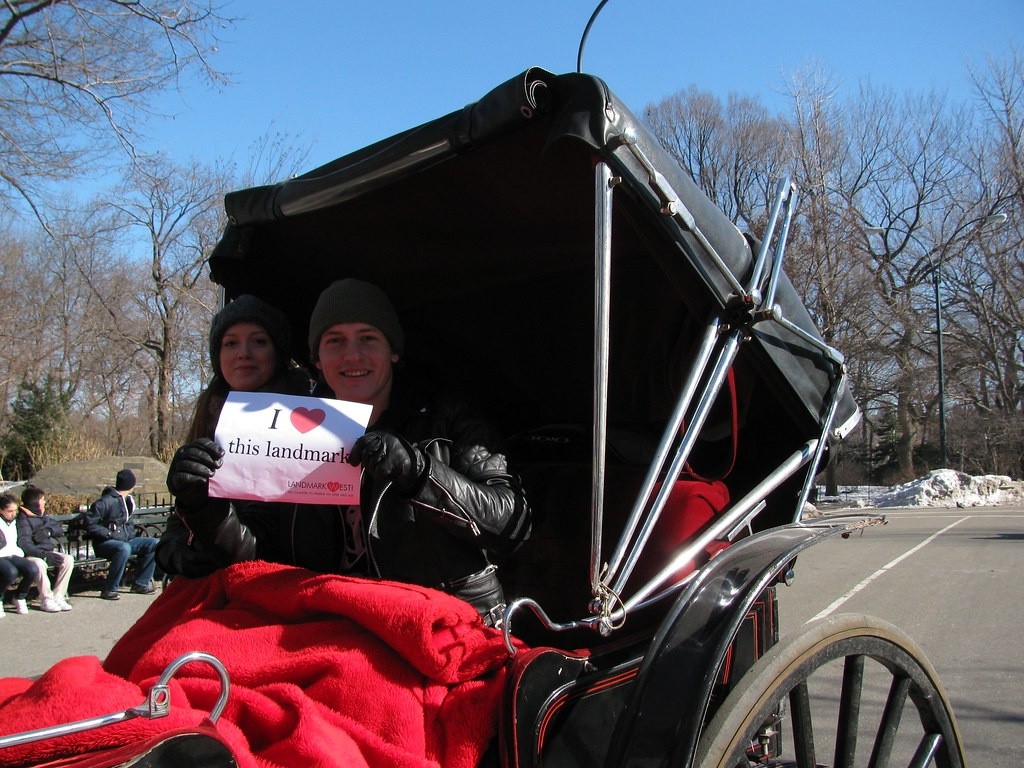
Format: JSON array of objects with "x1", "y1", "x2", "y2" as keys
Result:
[{"x1": 861, "y1": 212, "x2": 1009, "y2": 465}]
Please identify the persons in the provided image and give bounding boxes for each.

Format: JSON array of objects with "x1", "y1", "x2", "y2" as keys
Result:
[
  {"x1": 166, "y1": 281, "x2": 532, "y2": 633},
  {"x1": 85, "y1": 469, "x2": 160, "y2": 600},
  {"x1": 155, "y1": 295, "x2": 326, "y2": 576},
  {"x1": 0, "y1": 493, "x2": 40, "y2": 618},
  {"x1": 17, "y1": 488, "x2": 75, "y2": 612}
]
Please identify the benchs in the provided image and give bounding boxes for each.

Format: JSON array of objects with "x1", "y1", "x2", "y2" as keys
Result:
[
  {"x1": 0, "y1": 506, "x2": 175, "y2": 605},
  {"x1": 495, "y1": 481, "x2": 732, "y2": 768}
]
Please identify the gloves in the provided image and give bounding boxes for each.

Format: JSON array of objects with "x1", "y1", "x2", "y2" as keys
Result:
[
  {"x1": 166, "y1": 437, "x2": 224, "y2": 497},
  {"x1": 349, "y1": 430, "x2": 414, "y2": 485},
  {"x1": 42, "y1": 552, "x2": 63, "y2": 567},
  {"x1": 33, "y1": 526, "x2": 52, "y2": 542}
]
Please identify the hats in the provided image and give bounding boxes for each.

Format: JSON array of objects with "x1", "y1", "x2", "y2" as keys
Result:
[
  {"x1": 116, "y1": 470, "x2": 136, "y2": 488},
  {"x1": 210, "y1": 295, "x2": 292, "y2": 379},
  {"x1": 309, "y1": 278, "x2": 405, "y2": 357}
]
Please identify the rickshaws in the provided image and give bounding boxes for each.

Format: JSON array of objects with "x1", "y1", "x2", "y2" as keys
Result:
[{"x1": 0, "y1": 0, "x2": 976, "y2": 768}]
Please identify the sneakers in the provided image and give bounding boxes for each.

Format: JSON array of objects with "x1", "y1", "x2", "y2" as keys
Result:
[
  {"x1": 59, "y1": 598, "x2": 72, "y2": 611},
  {"x1": 40, "y1": 598, "x2": 60, "y2": 612},
  {"x1": 100, "y1": 588, "x2": 120, "y2": 600},
  {"x1": 0, "y1": 601, "x2": 6, "y2": 620},
  {"x1": 131, "y1": 583, "x2": 156, "y2": 595},
  {"x1": 11, "y1": 598, "x2": 29, "y2": 615}
]
